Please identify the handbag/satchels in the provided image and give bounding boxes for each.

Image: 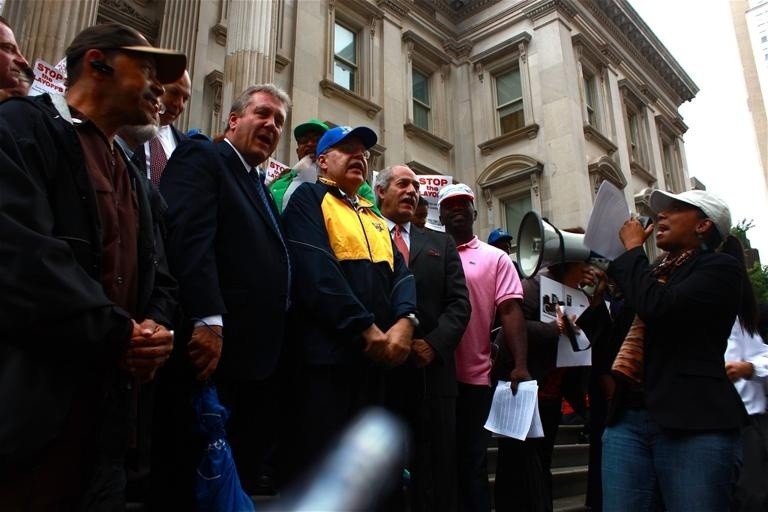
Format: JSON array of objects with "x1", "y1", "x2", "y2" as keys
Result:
[{"x1": 402, "y1": 311, "x2": 420, "y2": 326}]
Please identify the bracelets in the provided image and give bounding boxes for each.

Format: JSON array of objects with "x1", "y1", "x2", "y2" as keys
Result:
[{"x1": 402, "y1": 311, "x2": 420, "y2": 326}]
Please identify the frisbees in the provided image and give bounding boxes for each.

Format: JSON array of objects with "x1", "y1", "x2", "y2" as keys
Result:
[{"x1": 88, "y1": 58, "x2": 113, "y2": 78}]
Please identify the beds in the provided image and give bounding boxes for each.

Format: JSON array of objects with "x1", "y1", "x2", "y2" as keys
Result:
[{"x1": 183, "y1": 360, "x2": 257, "y2": 512}]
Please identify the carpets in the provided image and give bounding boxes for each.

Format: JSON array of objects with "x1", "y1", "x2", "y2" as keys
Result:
[
  {"x1": 149, "y1": 136, "x2": 168, "y2": 185},
  {"x1": 251, "y1": 167, "x2": 291, "y2": 310},
  {"x1": 394, "y1": 225, "x2": 410, "y2": 271}
]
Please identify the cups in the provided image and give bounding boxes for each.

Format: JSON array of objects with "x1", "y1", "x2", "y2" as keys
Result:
[
  {"x1": 158, "y1": 102, "x2": 166, "y2": 115},
  {"x1": 341, "y1": 143, "x2": 370, "y2": 159},
  {"x1": 298, "y1": 136, "x2": 318, "y2": 144}
]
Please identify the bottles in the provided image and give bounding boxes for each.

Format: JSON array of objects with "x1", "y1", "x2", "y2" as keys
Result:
[
  {"x1": 66, "y1": 23, "x2": 186, "y2": 85},
  {"x1": 316, "y1": 127, "x2": 377, "y2": 156},
  {"x1": 488, "y1": 229, "x2": 512, "y2": 243},
  {"x1": 187, "y1": 128, "x2": 213, "y2": 141},
  {"x1": 294, "y1": 119, "x2": 329, "y2": 137},
  {"x1": 437, "y1": 184, "x2": 475, "y2": 205},
  {"x1": 649, "y1": 189, "x2": 732, "y2": 242}
]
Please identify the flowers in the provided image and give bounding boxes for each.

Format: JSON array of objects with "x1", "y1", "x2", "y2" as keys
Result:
[{"x1": 635, "y1": 216, "x2": 653, "y2": 229}]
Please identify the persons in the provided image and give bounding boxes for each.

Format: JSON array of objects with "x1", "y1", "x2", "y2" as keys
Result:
[
  {"x1": 0, "y1": 23, "x2": 185, "y2": 512},
  {"x1": 158, "y1": 83, "x2": 284, "y2": 512},
  {"x1": 594, "y1": 190, "x2": 757, "y2": 511},
  {"x1": 370, "y1": 163, "x2": 473, "y2": 512},
  {"x1": 0, "y1": 18, "x2": 767, "y2": 512},
  {"x1": 435, "y1": 182, "x2": 533, "y2": 511},
  {"x1": 281, "y1": 123, "x2": 420, "y2": 511}
]
